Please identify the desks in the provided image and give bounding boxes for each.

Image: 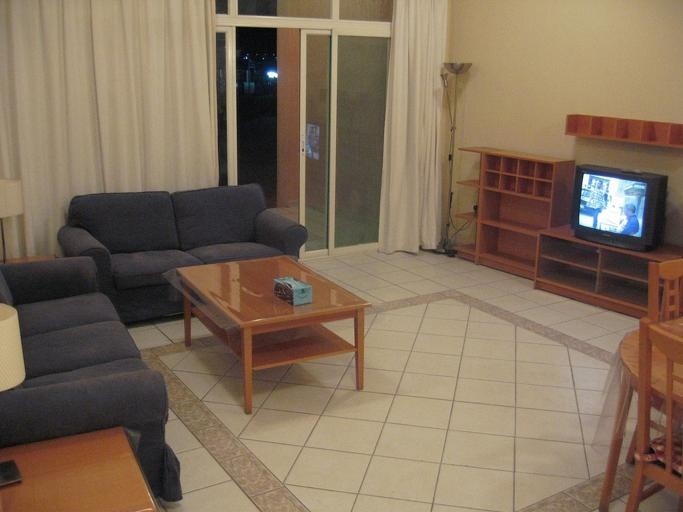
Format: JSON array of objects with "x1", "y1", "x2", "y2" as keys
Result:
[
  {"x1": 1, "y1": 256, "x2": 49, "y2": 264},
  {"x1": 599, "y1": 319, "x2": 683, "y2": 512}
]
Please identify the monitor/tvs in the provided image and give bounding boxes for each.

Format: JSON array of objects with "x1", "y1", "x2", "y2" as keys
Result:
[
  {"x1": 570, "y1": 164, "x2": 668, "y2": 252},
  {"x1": 306, "y1": 120, "x2": 326, "y2": 161}
]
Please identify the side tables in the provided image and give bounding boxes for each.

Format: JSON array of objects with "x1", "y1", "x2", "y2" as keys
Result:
[{"x1": 0, "y1": 426, "x2": 161, "y2": 511}]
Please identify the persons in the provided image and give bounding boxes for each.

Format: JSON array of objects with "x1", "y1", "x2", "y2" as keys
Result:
[{"x1": 616, "y1": 203, "x2": 639, "y2": 236}]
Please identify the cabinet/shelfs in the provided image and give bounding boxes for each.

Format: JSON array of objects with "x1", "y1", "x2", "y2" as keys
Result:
[
  {"x1": 534, "y1": 225, "x2": 683, "y2": 319},
  {"x1": 455, "y1": 146, "x2": 575, "y2": 281}
]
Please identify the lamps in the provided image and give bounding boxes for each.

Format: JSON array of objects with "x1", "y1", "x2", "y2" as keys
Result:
[
  {"x1": 0, "y1": 178, "x2": 23, "y2": 263},
  {"x1": 434, "y1": 61, "x2": 472, "y2": 256},
  {"x1": 0, "y1": 302, "x2": 27, "y2": 488}
]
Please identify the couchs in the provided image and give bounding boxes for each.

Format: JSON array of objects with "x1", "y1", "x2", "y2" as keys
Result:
[
  {"x1": 0, "y1": 256, "x2": 181, "y2": 502},
  {"x1": 57, "y1": 183, "x2": 308, "y2": 325}
]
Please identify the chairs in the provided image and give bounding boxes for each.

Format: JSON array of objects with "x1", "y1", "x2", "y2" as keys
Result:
[
  {"x1": 624, "y1": 258, "x2": 683, "y2": 462},
  {"x1": 627, "y1": 317, "x2": 682, "y2": 512}
]
